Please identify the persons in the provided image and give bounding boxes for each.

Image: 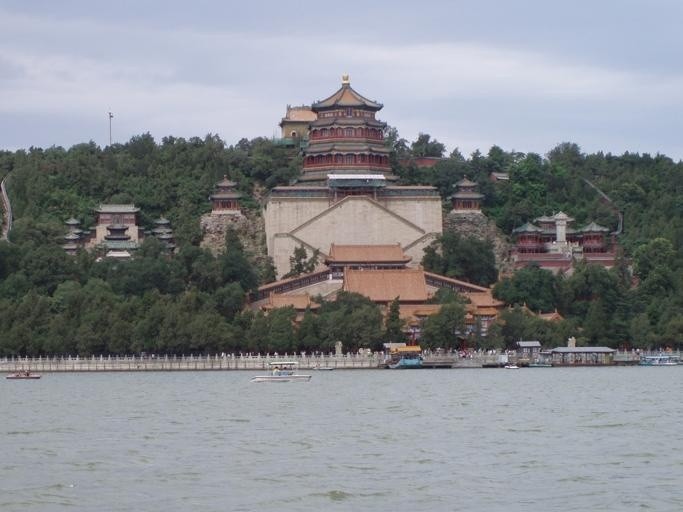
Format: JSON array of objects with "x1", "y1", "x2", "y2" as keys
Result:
[
  {"x1": 23, "y1": 370, "x2": 29, "y2": 376},
  {"x1": 272, "y1": 367, "x2": 280, "y2": 376},
  {"x1": 280, "y1": 367, "x2": 288, "y2": 376},
  {"x1": 17, "y1": 370, "x2": 21, "y2": 377},
  {"x1": 15, "y1": 345, "x2": 678, "y2": 368}
]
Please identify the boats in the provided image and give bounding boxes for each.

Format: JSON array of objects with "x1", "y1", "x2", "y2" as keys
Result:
[
  {"x1": 313, "y1": 365, "x2": 332, "y2": 371},
  {"x1": 6, "y1": 372, "x2": 40, "y2": 379},
  {"x1": 638, "y1": 354, "x2": 681, "y2": 366},
  {"x1": 504, "y1": 365, "x2": 518, "y2": 369},
  {"x1": 248, "y1": 361, "x2": 311, "y2": 382}
]
